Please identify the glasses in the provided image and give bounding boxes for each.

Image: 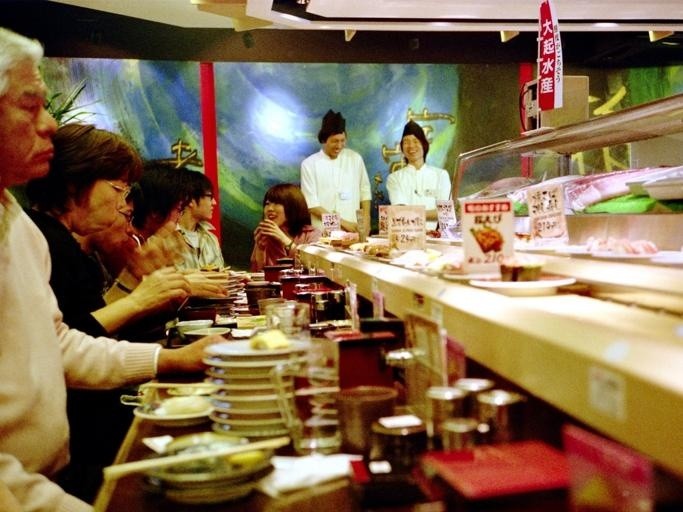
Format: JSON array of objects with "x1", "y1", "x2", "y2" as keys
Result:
[
  {"x1": 100, "y1": 178, "x2": 131, "y2": 199},
  {"x1": 119, "y1": 210, "x2": 137, "y2": 223},
  {"x1": 205, "y1": 193, "x2": 214, "y2": 200}
]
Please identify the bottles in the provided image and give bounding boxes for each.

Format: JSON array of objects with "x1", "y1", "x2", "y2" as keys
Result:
[{"x1": 368, "y1": 377, "x2": 528, "y2": 469}]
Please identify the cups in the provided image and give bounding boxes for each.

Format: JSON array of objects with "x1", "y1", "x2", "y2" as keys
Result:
[
  {"x1": 337, "y1": 383, "x2": 397, "y2": 455},
  {"x1": 244, "y1": 257, "x2": 355, "y2": 320}
]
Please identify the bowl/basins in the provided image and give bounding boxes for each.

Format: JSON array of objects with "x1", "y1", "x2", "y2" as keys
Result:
[
  {"x1": 175, "y1": 318, "x2": 230, "y2": 339},
  {"x1": 139, "y1": 432, "x2": 275, "y2": 505}
]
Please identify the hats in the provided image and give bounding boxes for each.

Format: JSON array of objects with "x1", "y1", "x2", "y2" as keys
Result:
[
  {"x1": 322, "y1": 108, "x2": 346, "y2": 131},
  {"x1": 403, "y1": 119, "x2": 425, "y2": 139}
]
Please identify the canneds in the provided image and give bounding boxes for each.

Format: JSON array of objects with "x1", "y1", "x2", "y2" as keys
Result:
[
  {"x1": 385, "y1": 350, "x2": 413, "y2": 405},
  {"x1": 369, "y1": 377, "x2": 529, "y2": 472}
]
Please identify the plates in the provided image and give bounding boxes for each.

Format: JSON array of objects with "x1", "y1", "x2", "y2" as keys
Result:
[
  {"x1": 132, "y1": 331, "x2": 309, "y2": 450},
  {"x1": 517, "y1": 234, "x2": 660, "y2": 262},
  {"x1": 314, "y1": 244, "x2": 578, "y2": 297},
  {"x1": 624, "y1": 164, "x2": 682, "y2": 201}
]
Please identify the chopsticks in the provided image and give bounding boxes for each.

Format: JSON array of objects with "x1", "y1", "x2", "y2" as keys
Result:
[{"x1": 102, "y1": 437, "x2": 290, "y2": 483}]
